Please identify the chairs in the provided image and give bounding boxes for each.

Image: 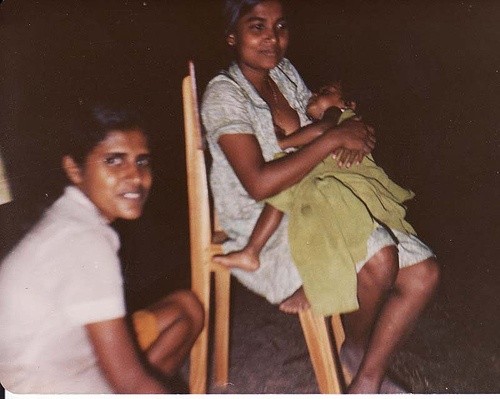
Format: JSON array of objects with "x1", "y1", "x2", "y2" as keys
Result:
[{"x1": 182, "y1": 57, "x2": 354, "y2": 394}]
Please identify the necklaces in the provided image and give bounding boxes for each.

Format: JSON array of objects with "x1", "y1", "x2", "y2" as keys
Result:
[{"x1": 257, "y1": 76, "x2": 279, "y2": 112}]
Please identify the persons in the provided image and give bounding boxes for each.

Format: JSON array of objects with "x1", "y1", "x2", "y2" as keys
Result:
[
  {"x1": 212, "y1": 77, "x2": 373, "y2": 315},
  {"x1": 200, "y1": 0, "x2": 443, "y2": 394},
  {"x1": 1, "y1": 96, "x2": 205, "y2": 393}
]
